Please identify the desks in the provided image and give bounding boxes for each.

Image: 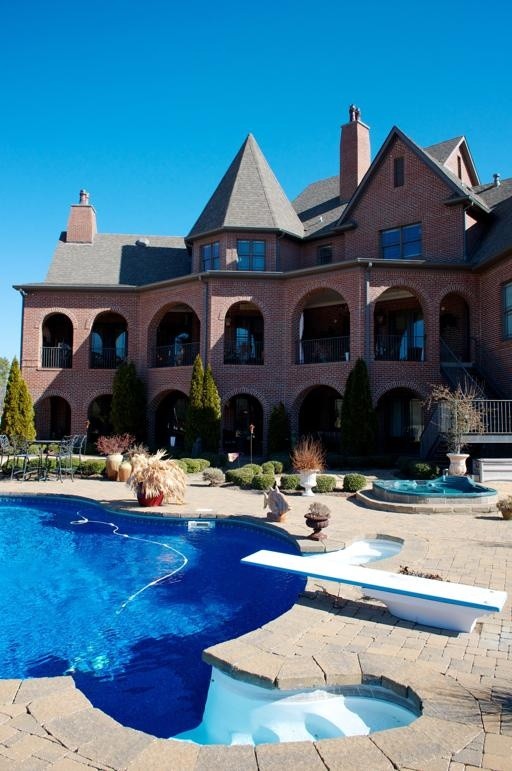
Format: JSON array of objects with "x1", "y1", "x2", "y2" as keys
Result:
[{"x1": 20, "y1": 439, "x2": 68, "y2": 481}]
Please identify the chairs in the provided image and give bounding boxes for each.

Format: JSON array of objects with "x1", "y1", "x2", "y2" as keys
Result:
[
  {"x1": 10, "y1": 434, "x2": 40, "y2": 482},
  {"x1": 64, "y1": 433, "x2": 87, "y2": 478},
  {"x1": 0, "y1": 435, "x2": 17, "y2": 479},
  {"x1": 45, "y1": 435, "x2": 79, "y2": 482}
]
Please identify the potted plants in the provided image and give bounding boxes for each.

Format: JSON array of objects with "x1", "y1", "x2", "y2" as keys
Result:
[
  {"x1": 124, "y1": 443, "x2": 168, "y2": 472},
  {"x1": 419, "y1": 381, "x2": 485, "y2": 477},
  {"x1": 304, "y1": 503, "x2": 331, "y2": 541},
  {"x1": 496, "y1": 493, "x2": 512, "y2": 519},
  {"x1": 130, "y1": 461, "x2": 187, "y2": 507},
  {"x1": 290, "y1": 436, "x2": 324, "y2": 497}
]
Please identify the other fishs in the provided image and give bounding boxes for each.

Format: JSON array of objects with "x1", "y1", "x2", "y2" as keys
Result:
[{"x1": 262, "y1": 485, "x2": 292, "y2": 515}]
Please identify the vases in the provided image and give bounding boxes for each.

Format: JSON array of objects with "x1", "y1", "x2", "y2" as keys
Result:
[
  {"x1": 106, "y1": 453, "x2": 123, "y2": 480},
  {"x1": 119, "y1": 460, "x2": 132, "y2": 481}
]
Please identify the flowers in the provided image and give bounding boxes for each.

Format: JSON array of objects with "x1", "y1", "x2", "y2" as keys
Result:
[{"x1": 95, "y1": 433, "x2": 136, "y2": 454}]
